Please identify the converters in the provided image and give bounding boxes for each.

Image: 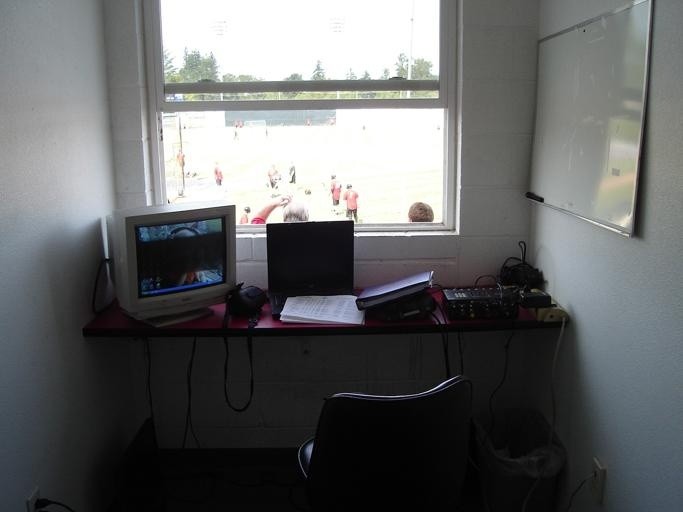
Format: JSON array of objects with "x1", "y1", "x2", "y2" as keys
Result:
[{"x1": 523, "y1": 292, "x2": 551, "y2": 307}]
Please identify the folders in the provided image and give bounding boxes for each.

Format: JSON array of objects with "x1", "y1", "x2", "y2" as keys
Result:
[{"x1": 355, "y1": 268, "x2": 433, "y2": 311}]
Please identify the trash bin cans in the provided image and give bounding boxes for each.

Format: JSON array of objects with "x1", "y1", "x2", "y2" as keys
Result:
[{"x1": 472, "y1": 405, "x2": 567, "y2": 512}]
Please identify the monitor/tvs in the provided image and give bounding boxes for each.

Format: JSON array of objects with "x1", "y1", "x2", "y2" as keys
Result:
[{"x1": 107, "y1": 201, "x2": 236, "y2": 329}]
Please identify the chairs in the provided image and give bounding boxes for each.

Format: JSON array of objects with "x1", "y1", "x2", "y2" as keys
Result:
[{"x1": 297, "y1": 376, "x2": 476, "y2": 511}]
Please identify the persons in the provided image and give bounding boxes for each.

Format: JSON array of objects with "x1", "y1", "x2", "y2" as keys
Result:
[
  {"x1": 232, "y1": 125, "x2": 239, "y2": 141},
  {"x1": 250, "y1": 193, "x2": 310, "y2": 225},
  {"x1": 342, "y1": 183, "x2": 359, "y2": 223},
  {"x1": 160, "y1": 229, "x2": 207, "y2": 289},
  {"x1": 213, "y1": 161, "x2": 223, "y2": 187},
  {"x1": 328, "y1": 174, "x2": 342, "y2": 216},
  {"x1": 239, "y1": 206, "x2": 250, "y2": 224},
  {"x1": 407, "y1": 201, "x2": 435, "y2": 222},
  {"x1": 264, "y1": 157, "x2": 296, "y2": 191}
]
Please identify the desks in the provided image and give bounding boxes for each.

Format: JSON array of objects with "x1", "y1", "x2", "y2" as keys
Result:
[{"x1": 81, "y1": 284, "x2": 569, "y2": 339}]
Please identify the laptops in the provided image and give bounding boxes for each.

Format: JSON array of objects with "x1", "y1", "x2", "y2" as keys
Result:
[{"x1": 267, "y1": 221, "x2": 361, "y2": 319}]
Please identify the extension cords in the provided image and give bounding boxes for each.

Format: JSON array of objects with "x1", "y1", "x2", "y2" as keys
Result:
[{"x1": 528, "y1": 288, "x2": 568, "y2": 322}]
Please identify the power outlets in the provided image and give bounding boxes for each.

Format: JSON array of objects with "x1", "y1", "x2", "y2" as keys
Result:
[
  {"x1": 26, "y1": 486, "x2": 40, "y2": 512},
  {"x1": 587, "y1": 456, "x2": 605, "y2": 506}
]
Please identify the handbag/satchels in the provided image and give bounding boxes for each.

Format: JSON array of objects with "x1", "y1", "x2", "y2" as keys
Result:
[{"x1": 223, "y1": 281, "x2": 267, "y2": 412}]
